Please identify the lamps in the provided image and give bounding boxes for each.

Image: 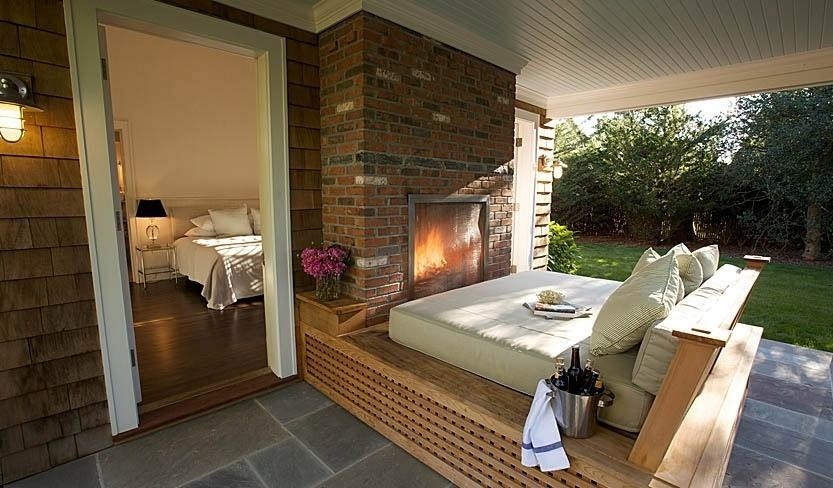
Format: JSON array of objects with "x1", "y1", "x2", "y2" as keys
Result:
[
  {"x1": 135, "y1": 198, "x2": 168, "y2": 250},
  {"x1": 0, "y1": 71, "x2": 43, "y2": 144},
  {"x1": 538, "y1": 153, "x2": 569, "y2": 179}
]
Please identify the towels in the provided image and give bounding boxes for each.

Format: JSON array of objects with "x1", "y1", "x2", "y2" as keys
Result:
[{"x1": 521, "y1": 380, "x2": 571, "y2": 473}]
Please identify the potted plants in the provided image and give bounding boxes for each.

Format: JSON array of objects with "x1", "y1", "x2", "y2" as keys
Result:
[{"x1": 299, "y1": 243, "x2": 355, "y2": 274}]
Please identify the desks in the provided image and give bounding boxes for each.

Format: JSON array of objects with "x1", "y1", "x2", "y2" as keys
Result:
[{"x1": 136, "y1": 243, "x2": 180, "y2": 290}]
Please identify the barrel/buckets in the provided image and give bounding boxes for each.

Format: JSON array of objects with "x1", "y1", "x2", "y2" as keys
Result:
[{"x1": 540, "y1": 372, "x2": 615, "y2": 439}]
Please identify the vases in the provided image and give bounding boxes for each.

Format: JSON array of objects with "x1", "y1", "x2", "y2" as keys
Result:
[{"x1": 315, "y1": 275, "x2": 342, "y2": 300}]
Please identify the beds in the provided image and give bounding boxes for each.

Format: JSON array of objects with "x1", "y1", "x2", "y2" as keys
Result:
[
  {"x1": 168, "y1": 205, "x2": 263, "y2": 311},
  {"x1": 294, "y1": 254, "x2": 771, "y2": 487}
]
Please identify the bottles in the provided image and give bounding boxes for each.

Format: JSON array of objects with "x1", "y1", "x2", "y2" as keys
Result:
[
  {"x1": 551, "y1": 358, "x2": 569, "y2": 391},
  {"x1": 582, "y1": 359, "x2": 599, "y2": 396},
  {"x1": 567, "y1": 345, "x2": 583, "y2": 395}
]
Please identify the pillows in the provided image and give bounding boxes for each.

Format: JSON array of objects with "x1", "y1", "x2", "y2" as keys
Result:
[
  {"x1": 184, "y1": 227, "x2": 216, "y2": 237},
  {"x1": 631, "y1": 244, "x2": 720, "y2": 304},
  {"x1": 207, "y1": 204, "x2": 253, "y2": 239},
  {"x1": 190, "y1": 215, "x2": 215, "y2": 231},
  {"x1": 250, "y1": 207, "x2": 262, "y2": 235},
  {"x1": 588, "y1": 251, "x2": 681, "y2": 357}
]
